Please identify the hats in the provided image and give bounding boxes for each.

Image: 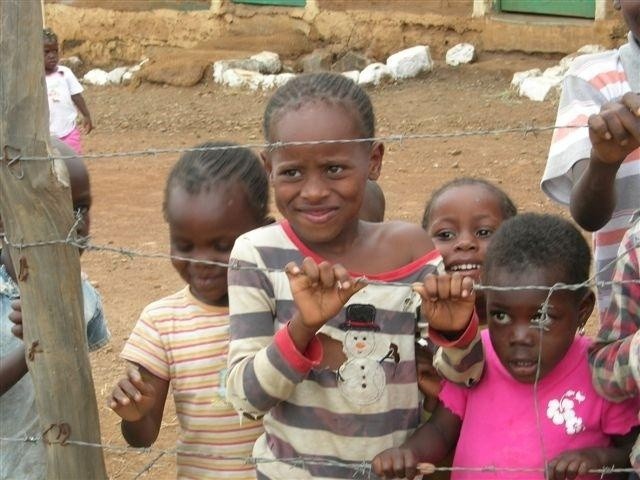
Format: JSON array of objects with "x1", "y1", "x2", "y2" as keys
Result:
[{"x1": 338, "y1": 304, "x2": 380, "y2": 333}]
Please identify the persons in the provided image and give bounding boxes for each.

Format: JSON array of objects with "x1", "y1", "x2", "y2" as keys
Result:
[
  {"x1": 539, "y1": 0, "x2": 639, "y2": 331},
  {"x1": 224, "y1": 73, "x2": 485, "y2": 480},
  {"x1": 417, "y1": 177, "x2": 519, "y2": 427},
  {"x1": 106, "y1": 140, "x2": 279, "y2": 480},
  {"x1": 370, "y1": 211, "x2": 639, "y2": 479},
  {"x1": 42, "y1": 28, "x2": 96, "y2": 156},
  {"x1": 588, "y1": 212, "x2": 638, "y2": 480},
  {"x1": 1, "y1": 133, "x2": 110, "y2": 480}
]
